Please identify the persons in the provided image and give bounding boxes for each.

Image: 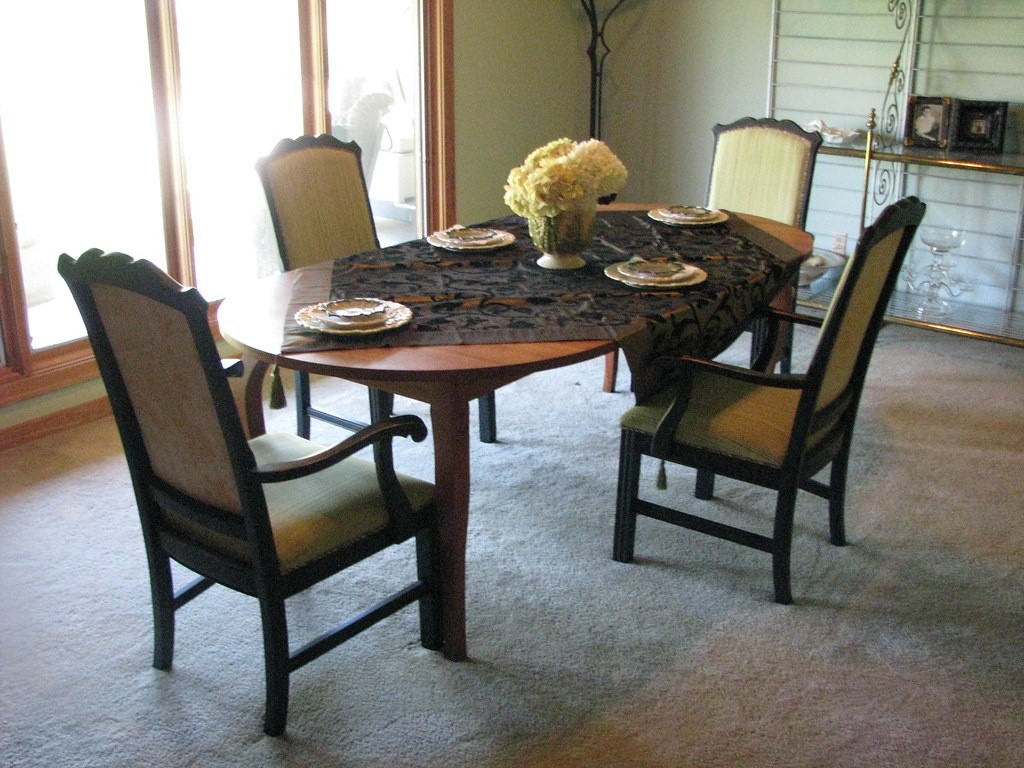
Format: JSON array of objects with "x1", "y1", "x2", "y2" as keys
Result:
[{"x1": 913, "y1": 106, "x2": 943, "y2": 142}]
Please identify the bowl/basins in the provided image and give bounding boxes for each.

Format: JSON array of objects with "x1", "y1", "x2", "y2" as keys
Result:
[{"x1": 798, "y1": 249, "x2": 846, "y2": 288}]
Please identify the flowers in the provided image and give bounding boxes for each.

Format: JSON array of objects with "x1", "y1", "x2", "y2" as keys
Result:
[{"x1": 503, "y1": 133, "x2": 630, "y2": 262}]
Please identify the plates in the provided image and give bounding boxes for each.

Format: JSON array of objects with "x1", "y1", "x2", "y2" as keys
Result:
[
  {"x1": 816, "y1": 127, "x2": 860, "y2": 144},
  {"x1": 446, "y1": 228, "x2": 496, "y2": 239},
  {"x1": 658, "y1": 209, "x2": 720, "y2": 221},
  {"x1": 307, "y1": 305, "x2": 390, "y2": 328},
  {"x1": 603, "y1": 263, "x2": 707, "y2": 290},
  {"x1": 435, "y1": 232, "x2": 504, "y2": 245},
  {"x1": 666, "y1": 205, "x2": 711, "y2": 216},
  {"x1": 319, "y1": 298, "x2": 389, "y2": 319},
  {"x1": 427, "y1": 231, "x2": 516, "y2": 252},
  {"x1": 625, "y1": 261, "x2": 683, "y2": 275},
  {"x1": 295, "y1": 300, "x2": 413, "y2": 335},
  {"x1": 647, "y1": 209, "x2": 728, "y2": 225},
  {"x1": 617, "y1": 265, "x2": 696, "y2": 282}
]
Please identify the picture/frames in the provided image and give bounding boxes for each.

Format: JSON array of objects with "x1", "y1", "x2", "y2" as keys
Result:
[
  {"x1": 902, "y1": 95, "x2": 952, "y2": 150},
  {"x1": 948, "y1": 99, "x2": 1009, "y2": 156}
]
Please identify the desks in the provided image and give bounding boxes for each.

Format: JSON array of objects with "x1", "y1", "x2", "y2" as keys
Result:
[{"x1": 216, "y1": 203, "x2": 816, "y2": 662}]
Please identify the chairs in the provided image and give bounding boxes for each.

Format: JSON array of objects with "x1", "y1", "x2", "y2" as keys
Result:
[
  {"x1": 603, "y1": 117, "x2": 822, "y2": 394},
  {"x1": 251, "y1": 132, "x2": 499, "y2": 446},
  {"x1": 610, "y1": 195, "x2": 927, "y2": 606},
  {"x1": 57, "y1": 248, "x2": 446, "y2": 734}
]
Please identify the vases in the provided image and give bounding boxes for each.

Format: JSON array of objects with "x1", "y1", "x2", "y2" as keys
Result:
[{"x1": 525, "y1": 196, "x2": 598, "y2": 270}]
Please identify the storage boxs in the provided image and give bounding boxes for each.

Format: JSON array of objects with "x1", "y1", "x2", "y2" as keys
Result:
[{"x1": 792, "y1": 130, "x2": 1024, "y2": 350}]
